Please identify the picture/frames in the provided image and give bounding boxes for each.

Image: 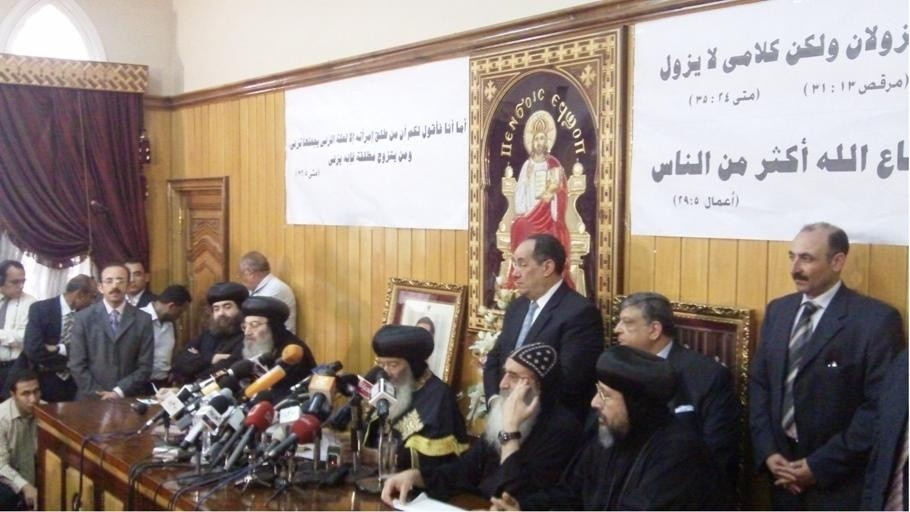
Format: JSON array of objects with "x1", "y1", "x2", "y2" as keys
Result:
[
  {"x1": 467, "y1": 25, "x2": 630, "y2": 348},
  {"x1": 373, "y1": 277, "x2": 468, "y2": 404}
]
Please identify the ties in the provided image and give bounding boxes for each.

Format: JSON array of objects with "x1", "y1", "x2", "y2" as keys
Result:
[
  {"x1": 18, "y1": 417, "x2": 35, "y2": 486},
  {"x1": 0, "y1": 296, "x2": 11, "y2": 329},
  {"x1": 56, "y1": 314, "x2": 73, "y2": 381},
  {"x1": 514, "y1": 302, "x2": 538, "y2": 348},
  {"x1": 783, "y1": 303, "x2": 817, "y2": 443},
  {"x1": 109, "y1": 311, "x2": 120, "y2": 330}
]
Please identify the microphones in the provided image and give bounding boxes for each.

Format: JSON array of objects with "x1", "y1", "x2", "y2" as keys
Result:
[{"x1": 136, "y1": 343, "x2": 395, "y2": 464}]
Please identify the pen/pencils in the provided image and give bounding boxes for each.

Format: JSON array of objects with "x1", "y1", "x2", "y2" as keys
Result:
[{"x1": 151, "y1": 382, "x2": 159, "y2": 396}]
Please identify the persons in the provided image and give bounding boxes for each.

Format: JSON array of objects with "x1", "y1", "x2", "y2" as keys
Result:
[
  {"x1": 480, "y1": 232, "x2": 605, "y2": 426},
  {"x1": 485, "y1": 343, "x2": 729, "y2": 511},
  {"x1": 337, "y1": 324, "x2": 469, "y2": 472},
  {"x1": 745, "y1": 220, "x2": 908, "y2": 511},
  {"x1": 378, "y1": 340, "x2": 584, "y2": 508},
  {"x1": 415, "y1": 316, "x2": 435, "y2": 336},
  {"x1": 498, "y1": 113, "x2": 577, "y2": 292},
  {"x1": 857, "y1": 350, "x2": 908, "y2": 510},
  {"x1": 0, "y1": 249, "x2": 319, "y2": 510}
]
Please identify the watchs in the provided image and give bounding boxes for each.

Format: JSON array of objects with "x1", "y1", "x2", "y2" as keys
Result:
[{"x1": 498, "y1": 427, "x2": 522, "y2": 448}]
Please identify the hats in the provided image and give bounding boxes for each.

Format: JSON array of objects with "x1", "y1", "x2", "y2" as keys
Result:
[{"x1": 511, "y1": 342, "x2": 559, "y2": 384}]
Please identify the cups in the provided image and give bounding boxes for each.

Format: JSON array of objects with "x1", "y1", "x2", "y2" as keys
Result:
[{"x1": 378, "y1": 436, "x2": 399, "y2": 477}]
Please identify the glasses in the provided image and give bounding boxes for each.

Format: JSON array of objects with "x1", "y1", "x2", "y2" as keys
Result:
[
  {"x1": 241, "y1": 322, "x2": 268, "y2": 328},
  {"x1": 594, "y1": 383, "x2": 623, "y2": 402}
]
